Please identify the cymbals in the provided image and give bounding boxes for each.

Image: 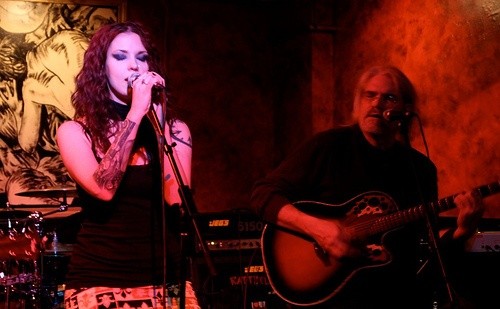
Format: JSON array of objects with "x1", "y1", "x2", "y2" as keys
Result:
[{"x1": 14, "y1": 184, "x2": 78, "y2": 199}]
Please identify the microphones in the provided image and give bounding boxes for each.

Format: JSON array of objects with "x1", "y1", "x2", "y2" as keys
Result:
[
  {"x1": 128, "y1": 72, "x2": 164, "y2": 91},
  {"x1": 383, "y1": 108, "x2": 412, "y2": 120}
]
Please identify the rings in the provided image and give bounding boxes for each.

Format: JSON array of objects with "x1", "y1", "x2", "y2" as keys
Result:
[{"x1": 142, "y1": 80, "x2": 149, "y2": 85}]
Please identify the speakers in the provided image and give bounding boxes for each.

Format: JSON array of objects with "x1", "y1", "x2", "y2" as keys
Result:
[
  {"x1": 189, "y1": 251, "x2": 290, "y2": 309},
  {"x1": 439, "y1": 250, "x2": 500, "y2": 309}
]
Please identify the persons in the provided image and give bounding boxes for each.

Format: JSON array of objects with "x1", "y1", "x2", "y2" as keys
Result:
[
  {"x1": 54, "y1": 21, "x2": 191, "y2": 309},
  {"x1": 249, "y1": 64, "x2": 485, "y2": 309}
]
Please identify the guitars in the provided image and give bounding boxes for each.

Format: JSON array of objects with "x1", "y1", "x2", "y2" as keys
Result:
[{"x1": 260, "y1": 181, "x2": 500, "y2": 305}]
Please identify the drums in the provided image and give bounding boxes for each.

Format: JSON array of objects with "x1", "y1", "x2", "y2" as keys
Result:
[
  {"x1": 0, "y1": 208, "x2": 42, "y2": 309},
  {"x1": 42, "y1": 242, "x2": 72, "y2": 297}
]
